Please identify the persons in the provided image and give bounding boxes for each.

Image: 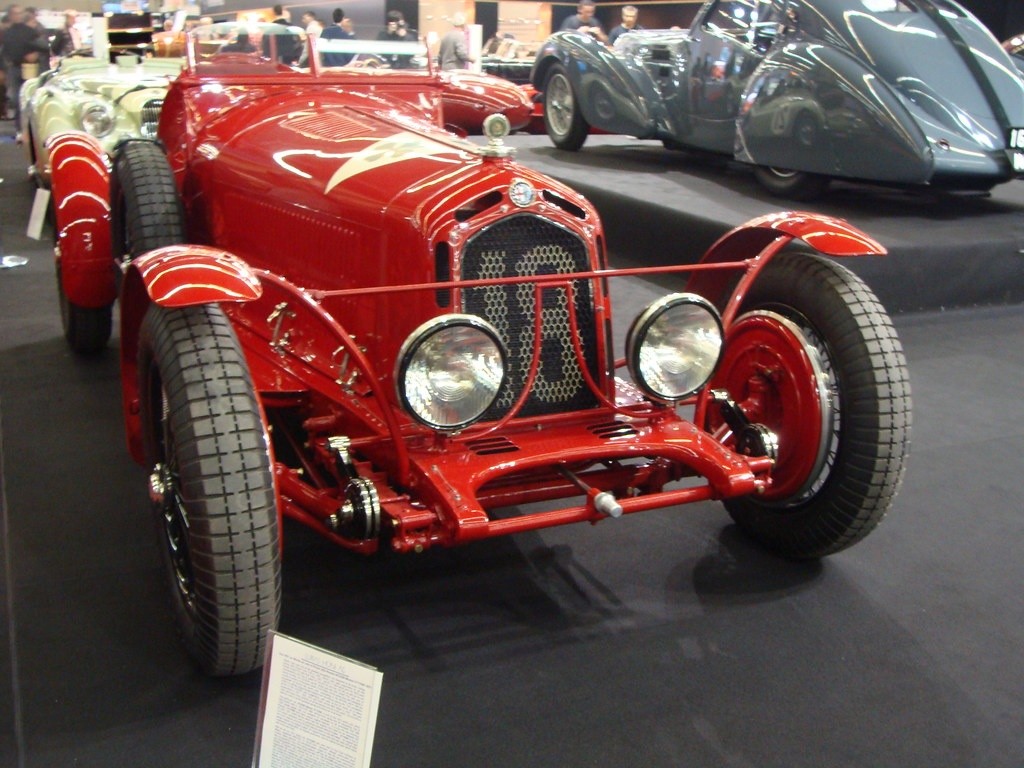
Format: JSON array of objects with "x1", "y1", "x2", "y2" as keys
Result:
[
  {"x1": 0, "y1": 2, "x2": 473, "y2": 118},
  {"x1": 560, "y1": 0, "x2": 608, "y2": 46},
  {"x1": 2, "y1": 4, "x2": 40, "y2": 142},
  {"x1": 607, "y1": 4, "x2": 645, "y2": 46}
]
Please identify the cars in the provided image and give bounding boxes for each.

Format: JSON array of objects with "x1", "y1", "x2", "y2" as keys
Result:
[
  {"x1": 28, "y1": 20, "x2": 913, "y2": 675},
  {"x1": 20, "y1": 46, "x2": 188, "y2": 229},
  {"x1": 530, "y1": 0, "x2": 1024, "y2": 202},
  {"x1": 151, "y1": 20, "x2": 621, "y2": 137}
]
